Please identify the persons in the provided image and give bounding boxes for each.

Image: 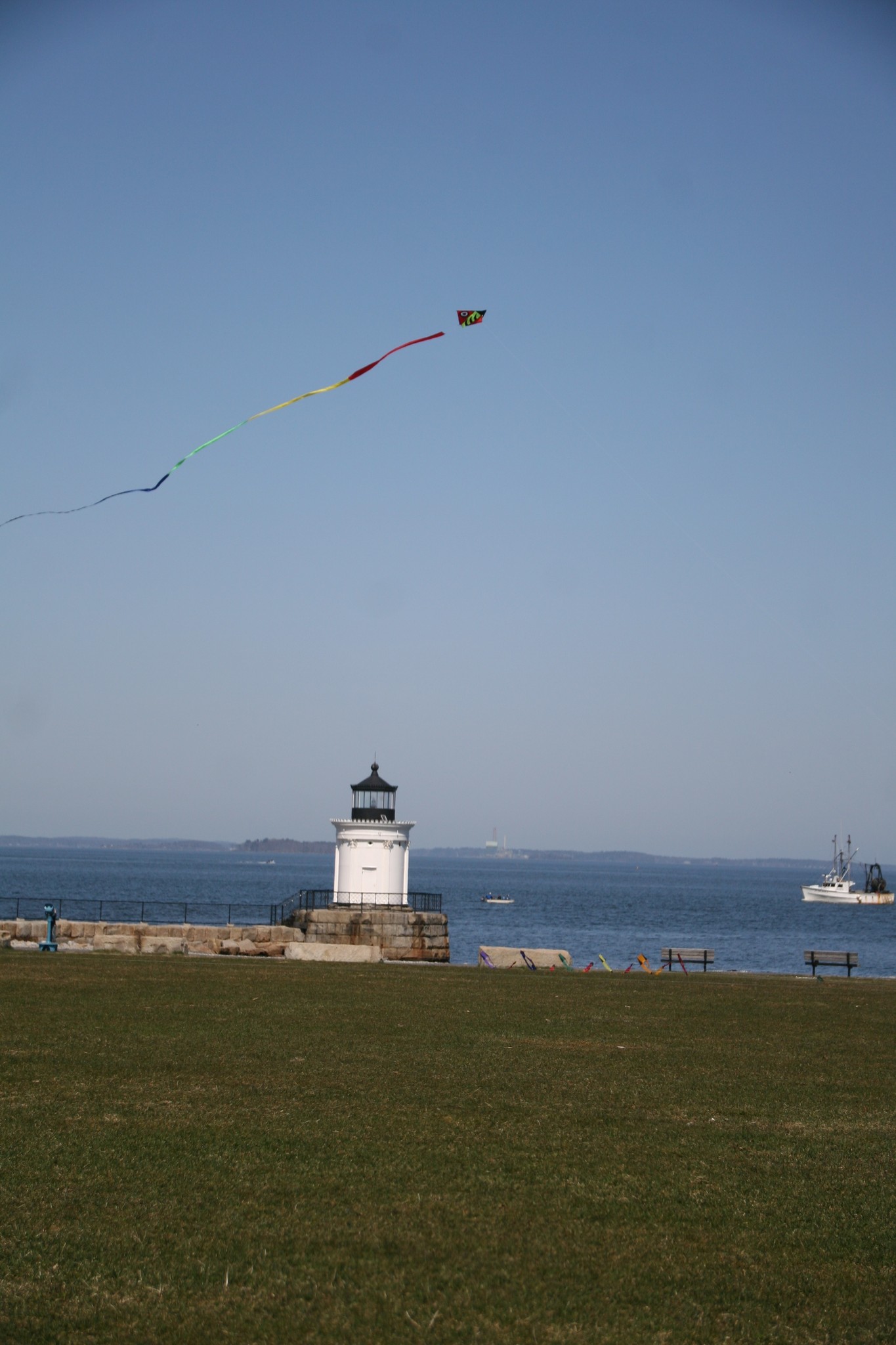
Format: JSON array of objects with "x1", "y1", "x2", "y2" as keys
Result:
[{"x1": 486, "y1": 892, "x2": 509, "y2": 900}]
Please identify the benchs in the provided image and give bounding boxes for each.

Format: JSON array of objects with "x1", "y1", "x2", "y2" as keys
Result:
[
  {"x1": 804, "y1": 949, "x2": 861, "y2": 977},
  {"x1": 661, "y1": 947, "x2": 715, "y2": 972}
]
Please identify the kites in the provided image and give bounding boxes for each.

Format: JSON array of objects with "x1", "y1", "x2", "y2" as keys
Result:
[{"x1": 0, "y1": 309, "x2": 486, "y2": 528}]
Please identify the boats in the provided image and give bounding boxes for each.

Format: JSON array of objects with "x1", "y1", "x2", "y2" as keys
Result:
[
  {"x1": 800, "y1": 834, "x2": 895, "y2": 905},
  {"x1": 482, "y1": 898, "x2": 516, "y2": 904}
]
